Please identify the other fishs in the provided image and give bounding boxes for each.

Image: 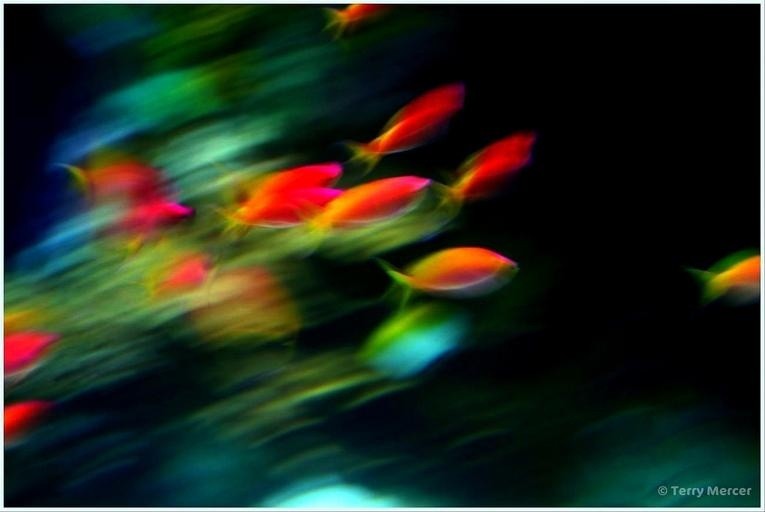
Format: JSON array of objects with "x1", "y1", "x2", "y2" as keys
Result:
[{"x1": 54, "y1": 155, "x2": 299, "y2": 344}]
